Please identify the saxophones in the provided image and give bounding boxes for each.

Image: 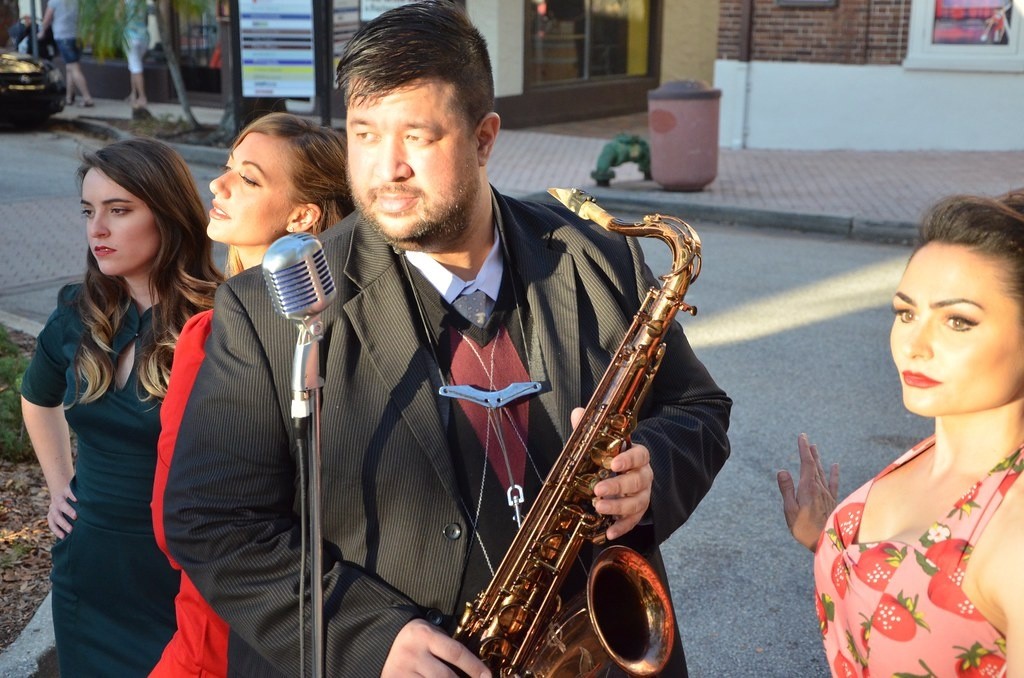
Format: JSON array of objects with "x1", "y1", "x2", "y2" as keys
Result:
[{"x1": 450, "y1": 187, "x2": 703, "y2": 678}]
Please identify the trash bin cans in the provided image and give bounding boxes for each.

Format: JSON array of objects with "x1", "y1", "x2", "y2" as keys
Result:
[{"x1": 646, "y1": 78, "x2": 722, "y2": 193}]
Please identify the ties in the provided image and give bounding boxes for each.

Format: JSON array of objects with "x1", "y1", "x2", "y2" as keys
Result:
[{"x1": 446, "y1": 292, "x2": 532, "y2": 507}]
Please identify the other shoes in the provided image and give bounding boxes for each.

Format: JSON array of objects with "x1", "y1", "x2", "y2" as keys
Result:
[{"x1": 134, "y1": 100, "x2": 148, "y2": 108}]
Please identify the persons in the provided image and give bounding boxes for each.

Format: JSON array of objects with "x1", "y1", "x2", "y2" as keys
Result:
[
  {"x1": 163, "y1": 0, "x2": 741, "y2": 678},
  {"x1": 15, "y1": 135, "x2": 230, "y2": 678},
  {"x1": 111, "y1": 0, "x2": 157, "y2": 109},
  {"x1": 35, "y1": 0, "x2": 99, "y2": 109},
  {"x1": 139, "y1": 105, "x2": 359, "y2": 678},
  {"x1": 776, "y1": 184, "x2": 1023, "y2": 676}
]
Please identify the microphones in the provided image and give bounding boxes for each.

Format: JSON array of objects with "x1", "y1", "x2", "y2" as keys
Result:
[{"x1": 259, "y1": 233, "x2": 338, "y2": 420}]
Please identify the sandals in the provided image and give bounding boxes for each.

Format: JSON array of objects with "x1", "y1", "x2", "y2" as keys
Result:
[
  {"x1": 78, "y1": 98, "x2": 95, "y2": 107},
  {"x1": 63, "y1": 97, "x2": 73, "y2": 105}
]
